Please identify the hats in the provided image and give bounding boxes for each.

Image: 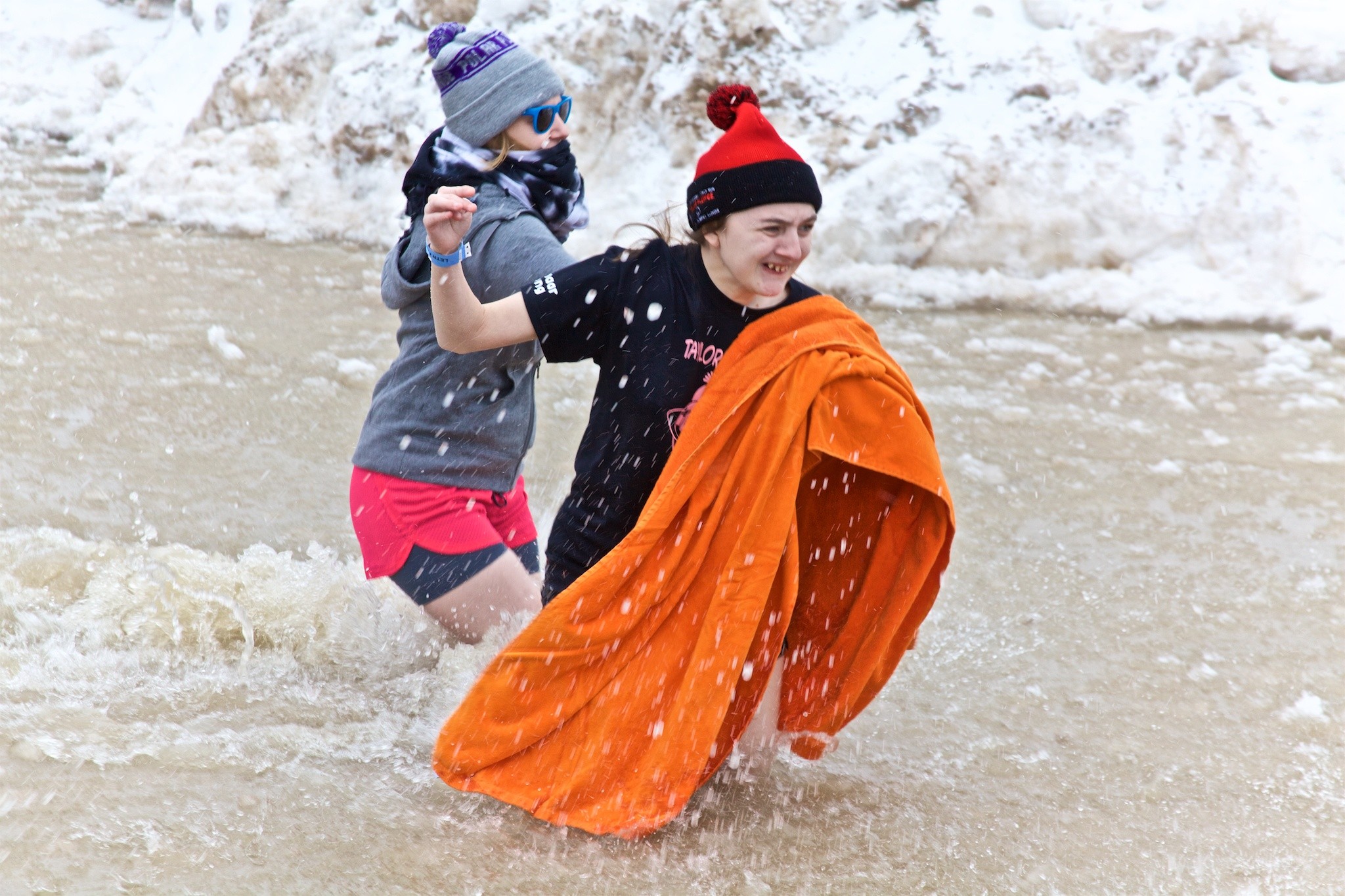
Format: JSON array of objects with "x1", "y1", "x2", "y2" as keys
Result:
[
  {"x1": 687, "y1": 82, "x2": 821, "y2": 230},
  {"x1": 425, "y1": 22, "x2": 565, "y2": 146}
]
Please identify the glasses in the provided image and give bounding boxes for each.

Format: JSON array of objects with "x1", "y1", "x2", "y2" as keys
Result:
[{"x1": 521, "y1": 95, "x2": 571, "y2": 133}]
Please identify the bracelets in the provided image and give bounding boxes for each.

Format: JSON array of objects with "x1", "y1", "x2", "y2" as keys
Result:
[{"x1": 425, "y1": 240, "x2": 473, "y2": 267}]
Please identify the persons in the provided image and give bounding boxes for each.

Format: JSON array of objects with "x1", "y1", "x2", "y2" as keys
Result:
[
  {"x1": 350, "y1": 23, "x2": 588, "y2": 641},
  {"x1": 423, "y1": 82, "x2": 823, "y2": 743}
]
